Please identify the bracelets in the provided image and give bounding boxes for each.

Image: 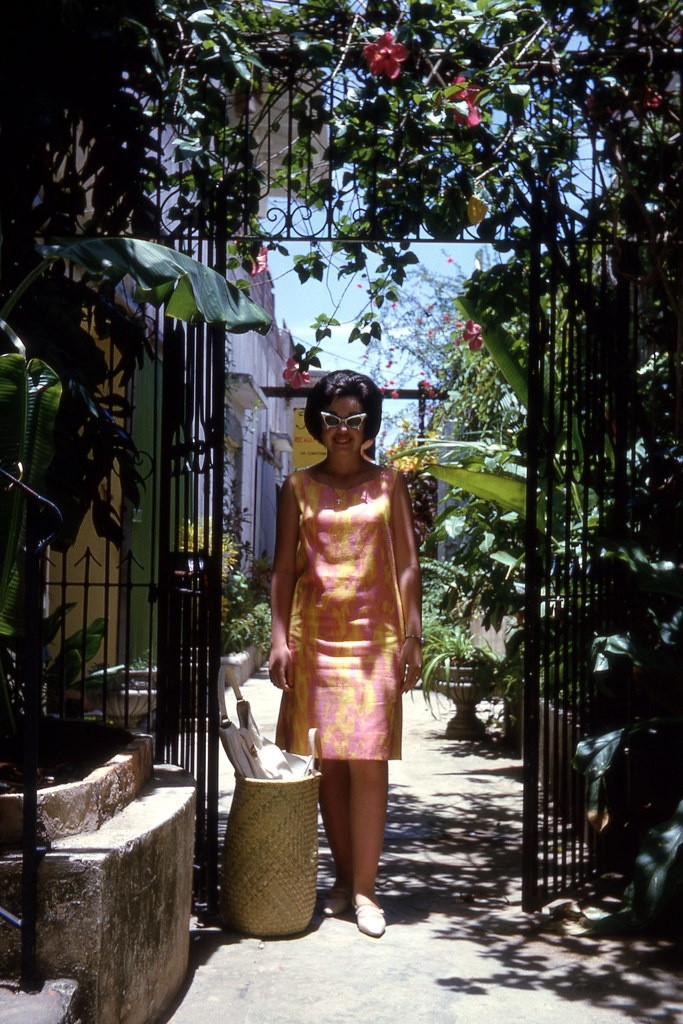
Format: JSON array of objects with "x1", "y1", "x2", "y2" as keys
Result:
[{"x1": 405, "y1": 635, "x2": 421, "y2": 644}]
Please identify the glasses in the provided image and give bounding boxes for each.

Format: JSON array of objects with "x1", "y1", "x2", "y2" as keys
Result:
[{"x1": 321, "y1": 411, "x2": 367, "y2": 429}]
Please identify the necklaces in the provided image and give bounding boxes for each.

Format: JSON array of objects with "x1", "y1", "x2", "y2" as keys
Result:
[{"x1": 325, "y1": 460, "x2": 365, "y2": 505}]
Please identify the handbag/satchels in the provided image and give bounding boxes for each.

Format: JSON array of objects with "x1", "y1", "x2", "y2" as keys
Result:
[{"x1": 219, "y1": 665, "x2": 314, "y2": 780}]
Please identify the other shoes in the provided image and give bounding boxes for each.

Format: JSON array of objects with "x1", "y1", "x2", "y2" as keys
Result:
[
  {"x1": 352, "y1": 891, "x2": 386, "y2": 938},
  {"x1": 322, "y1": 887, "x2": 352, "y2": 917}
]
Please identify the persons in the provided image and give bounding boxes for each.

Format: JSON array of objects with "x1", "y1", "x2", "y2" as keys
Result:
[{"x1": 269, "y1": 369, "x2": 423, "y2": 938}]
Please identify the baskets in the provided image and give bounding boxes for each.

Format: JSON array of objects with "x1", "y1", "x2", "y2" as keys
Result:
[{"x1": 219, "y1": 770, "x2": 323, "y2": 938}]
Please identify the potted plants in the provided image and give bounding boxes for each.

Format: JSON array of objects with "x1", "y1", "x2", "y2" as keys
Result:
[
  {"x1": 100, "y1": 681, "x2": 158, "y2": 735},
  {"x1": 97, "y1": 649, "x2": 156, "y2": 689},
  {"x1": 422, "y1": 630, "x2": 498, "y2": 739}
]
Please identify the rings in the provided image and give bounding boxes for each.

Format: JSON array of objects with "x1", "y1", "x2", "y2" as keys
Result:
[{"x1": 416, "y1": 676, "x2": 419, "y2": 679}]
